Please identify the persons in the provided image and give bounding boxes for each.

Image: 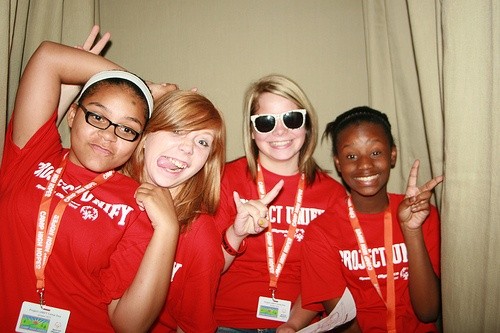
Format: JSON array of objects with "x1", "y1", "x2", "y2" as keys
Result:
[
  {"x1": 213, "y1": 75, "x2": 348, "y2": 333},
  {"x1": 55, "y1": 25, "x2": 225, "y2": 333},
  {"x1": 302, "y1": 106, "x2": 443, "y2": 333},
  {"x1": 0, "y1": 41, "x2": 198, "y2": 333}
]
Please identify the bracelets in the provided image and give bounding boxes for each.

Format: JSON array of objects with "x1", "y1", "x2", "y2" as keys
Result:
[{"x1": 222, "y1": 229, "x2": 246, "y2": 256}]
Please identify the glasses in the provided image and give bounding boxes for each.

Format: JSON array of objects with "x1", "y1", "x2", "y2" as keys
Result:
[
  {"x1": 78, "y1": 102, "x2": 144, "y2": 143},
  {"x1": 250, "y1": 108, "x2": 308, "y2": 135}
]
